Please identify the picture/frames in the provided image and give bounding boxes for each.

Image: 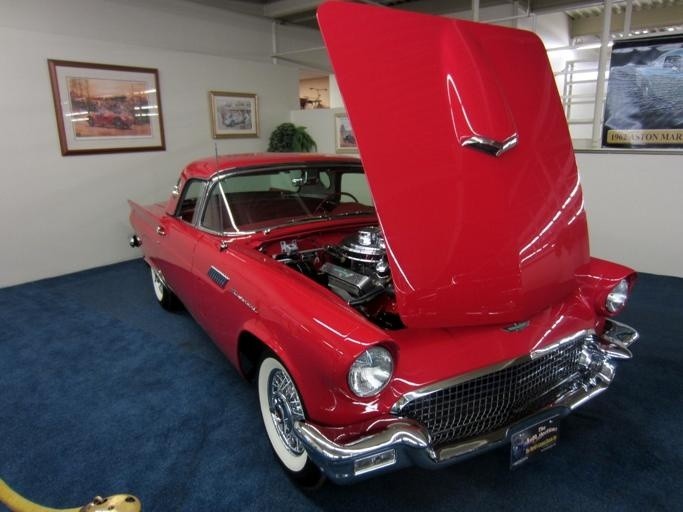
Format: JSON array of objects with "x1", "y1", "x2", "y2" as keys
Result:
[
  {"x1": 208, "y1": 89, "x2": 260, "y2": 139},
  {"x1": 47, "y1": 58, "x2": 166, "y2": 156},
  {"x1": 334, "y1": 112, "x2": 360, "y2": 155}
]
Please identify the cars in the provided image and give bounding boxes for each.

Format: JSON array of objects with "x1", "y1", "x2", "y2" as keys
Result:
[
  {"x1": 127, "y1": 2, "x2": 643, "y2": 482},
  {"x1": 86, "y1": 104, "x2": 136, "y2": 129}
]
